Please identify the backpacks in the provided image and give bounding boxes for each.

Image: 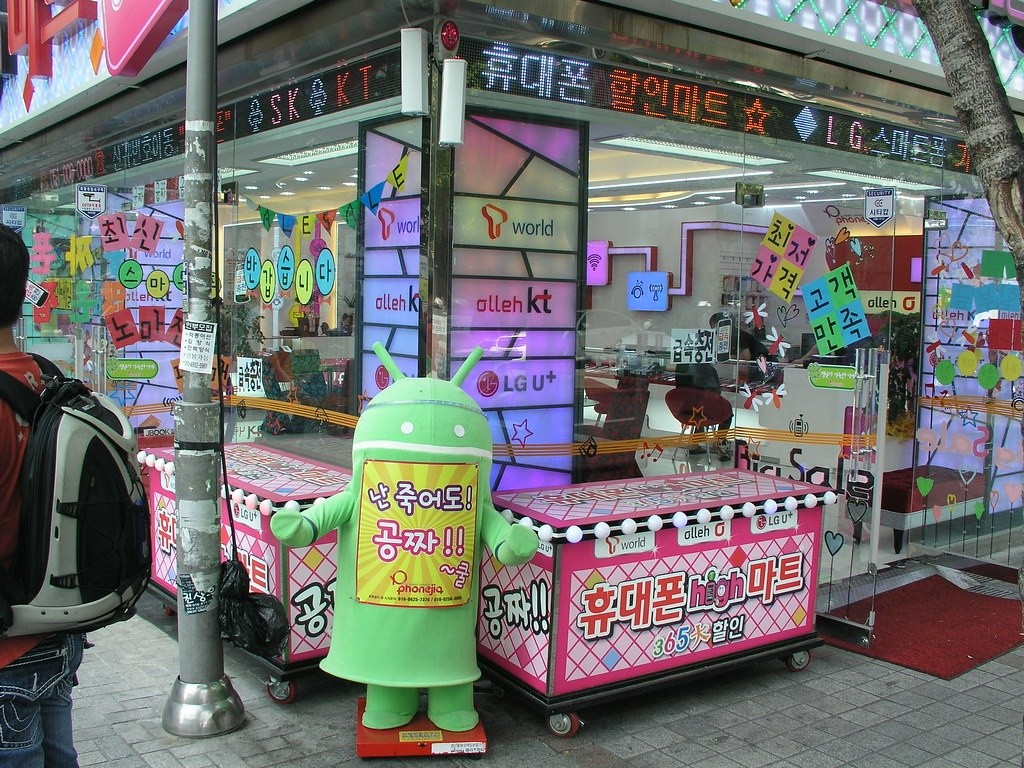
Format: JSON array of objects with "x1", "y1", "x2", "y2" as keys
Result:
[{"x1": 0, "y1": 352, "x2": 153, "y2": 640}]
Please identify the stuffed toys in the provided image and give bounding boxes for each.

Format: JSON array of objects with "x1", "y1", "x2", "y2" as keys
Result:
[{"x1": 269, "y1": 343, "x2": 540, "y2": 733}]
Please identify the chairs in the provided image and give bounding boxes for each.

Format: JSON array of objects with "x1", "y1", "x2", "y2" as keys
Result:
[
  {"x1": 665, "y1": 387, "x2": 732, "y2": 464},
  {"x1": 573, "y1": 375, "x2": 651, "y2": 482}
]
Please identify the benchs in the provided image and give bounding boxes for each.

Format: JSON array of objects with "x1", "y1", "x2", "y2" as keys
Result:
[{"x1": 851, "y1": 463, "x2": 987, "y2": 554}]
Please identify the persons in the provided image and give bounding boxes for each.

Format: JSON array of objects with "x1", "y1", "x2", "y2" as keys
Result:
[
  {"x1": 675, "y1": 364, "x2": 732, "y2": 463},
  {"x1": 709, "y1": 313, "x2": 785, "y2": 390},
  {"x1": 0, "y1": 223, "x2": 83, "y2": 768}
]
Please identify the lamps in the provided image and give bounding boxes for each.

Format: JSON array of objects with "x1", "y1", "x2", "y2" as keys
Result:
[
  {"x1": 399, "y1": 27, "x2": 435, "y2": 120},
  {"x1": 924, "y1": 218, "x2": 949, "y2": 232},
  {"x1": 437, "y1": 57, "x2": 468, "y2": 147}
]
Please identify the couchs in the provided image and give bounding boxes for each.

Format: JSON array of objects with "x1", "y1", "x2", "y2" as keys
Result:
[{"x1": 254, "y1": 348, "x2": 355, "y2": 436}]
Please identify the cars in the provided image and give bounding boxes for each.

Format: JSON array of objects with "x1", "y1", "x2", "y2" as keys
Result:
[{"x1": 488, "y1": 307, "x2": 677, "y2": 377}]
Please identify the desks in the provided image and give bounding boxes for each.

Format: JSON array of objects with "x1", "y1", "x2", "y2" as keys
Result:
[{"x1": 288, "y1": 365, "x2": 346, "y2": 396}]
